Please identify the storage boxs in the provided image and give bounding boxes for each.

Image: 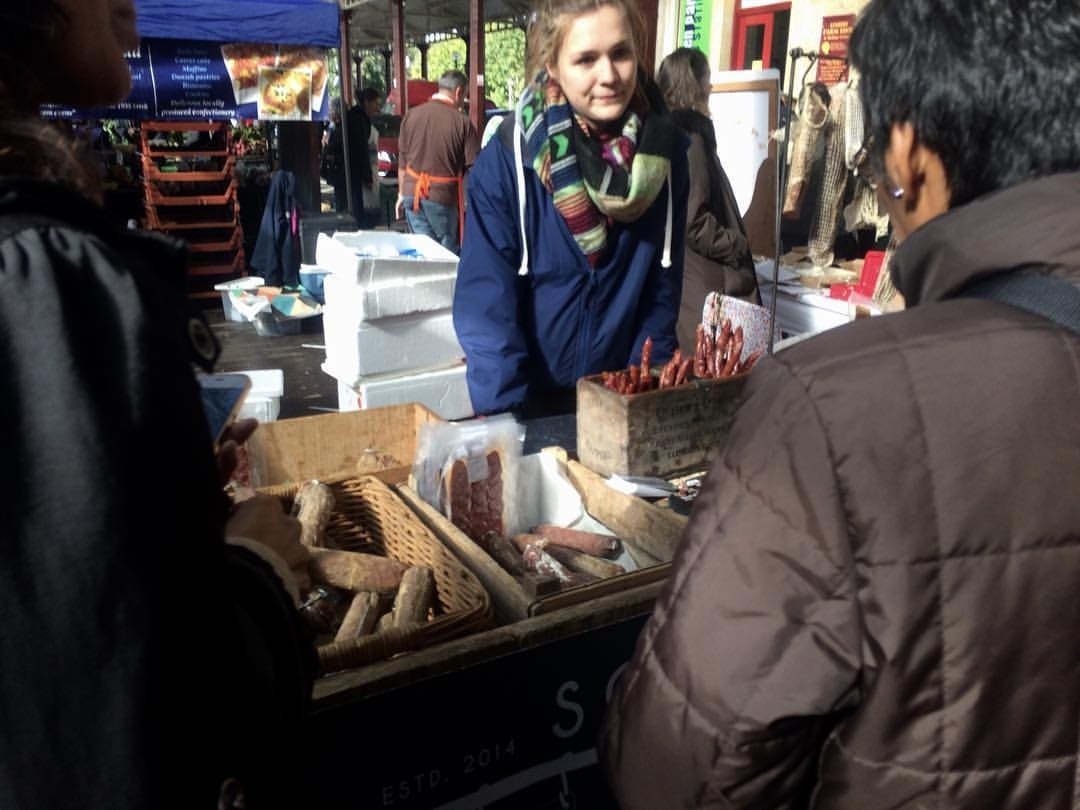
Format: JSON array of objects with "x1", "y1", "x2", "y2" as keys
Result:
[{"x1": 158, "y1": 228, "x2": 895, "y2": 810}]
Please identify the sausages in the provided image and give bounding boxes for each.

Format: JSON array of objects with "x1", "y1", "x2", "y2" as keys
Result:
[{"x1": 602, "y1": 314, "x2": 764, "y2": 406}]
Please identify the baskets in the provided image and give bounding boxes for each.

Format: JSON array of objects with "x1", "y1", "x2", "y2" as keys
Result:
[{"x1": 225, "y1": 473, "x2": 494, "y2": 681}]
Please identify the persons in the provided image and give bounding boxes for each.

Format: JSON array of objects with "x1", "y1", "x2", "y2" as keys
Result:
[
  {"x1": 69, "y1": 87, "x2": 384, "y2": 230},
  {"x1": 0, "y1": 1, "x2": 310, "y2": 810},
  {"x1": 396, "y1": 70, "x2": 482, "y2": 258},
  {"x1": 653, "y1": 46, "x2": 765, "y2": 357},
  {"x1": 448, "y1": 0, "x2": 692, "y2": 424},
  {"x1": 591, "y1": 1, "x2": 1080, "y2": 810}
]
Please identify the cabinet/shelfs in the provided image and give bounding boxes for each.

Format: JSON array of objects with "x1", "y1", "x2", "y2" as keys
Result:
[{"x1": 132, "y1": 118, "x2": 255, "y2": 311}]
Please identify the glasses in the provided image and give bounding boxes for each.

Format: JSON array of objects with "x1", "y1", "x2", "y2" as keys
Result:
[{"x1": 852, "y1": 125, "x2": 892, "y2": 191}]
